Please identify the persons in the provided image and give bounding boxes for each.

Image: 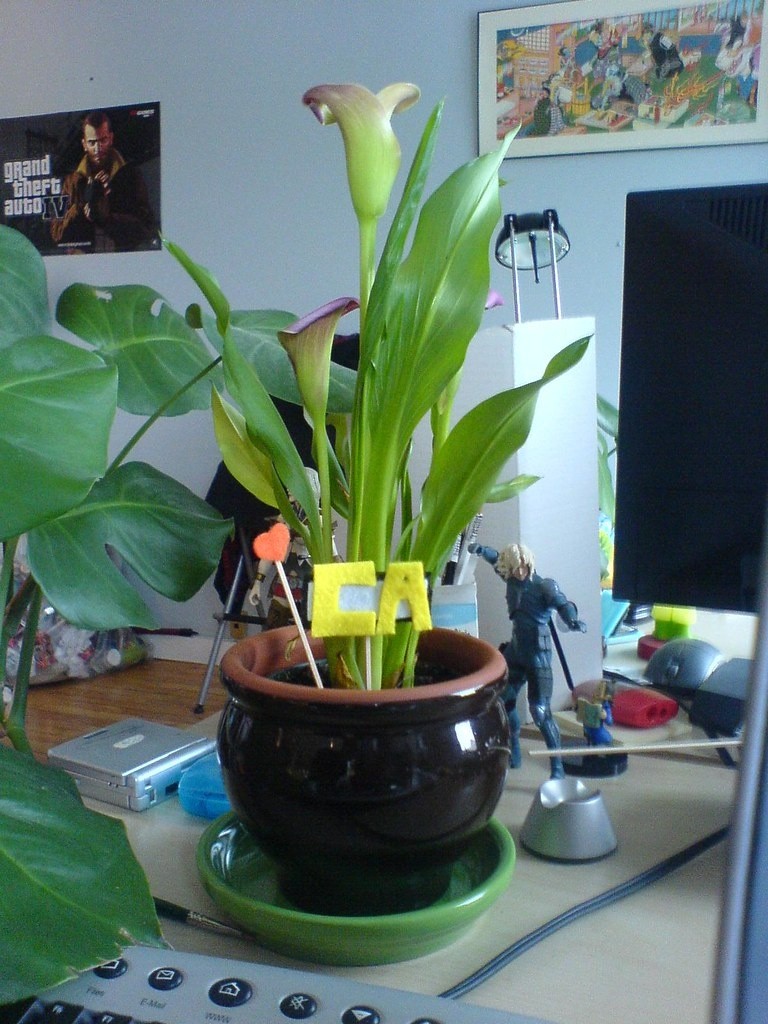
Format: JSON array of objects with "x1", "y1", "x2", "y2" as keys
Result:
[
  {"x1": 467, "y1": 541, "x2": 587, "y2": 781},
  {"x1": 575, "y1": 681, "x2": 614, "y2": 747}
]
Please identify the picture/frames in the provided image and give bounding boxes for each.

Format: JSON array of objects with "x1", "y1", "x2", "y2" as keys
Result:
[{"x1": 477, "y1": 0, "x2": 768, "y2": 160}]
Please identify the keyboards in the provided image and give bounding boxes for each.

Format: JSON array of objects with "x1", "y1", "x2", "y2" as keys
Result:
[{"x1": 0, "y1": 943, "x2": 565, "y2": 1024}]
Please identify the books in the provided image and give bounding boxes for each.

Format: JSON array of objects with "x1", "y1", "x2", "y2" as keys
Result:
[{"x1": 576, "y1": 697, "x2": 602, "y2": 728}]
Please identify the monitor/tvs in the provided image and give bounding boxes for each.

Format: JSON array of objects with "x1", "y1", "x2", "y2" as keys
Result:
[{"x1": 613, "y1": 182, "x2": 768, "y2": 737}]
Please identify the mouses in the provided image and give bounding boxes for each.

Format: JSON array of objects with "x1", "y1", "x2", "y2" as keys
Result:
[{"x1": 643, "y1": 638, "x2": 725, "y2": 690}]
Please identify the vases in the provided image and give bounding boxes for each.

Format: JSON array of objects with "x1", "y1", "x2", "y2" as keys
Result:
[
  {"x1": 160, "y1": 80, "x2": 602, "y2": 688},
  {"x1": 197, "y1": 620, "x2": 518, "y2": 968}
]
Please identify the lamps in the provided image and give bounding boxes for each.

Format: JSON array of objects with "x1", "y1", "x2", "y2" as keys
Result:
[{"x1": 493, "y1": 209, "x2": 570, "y2": 323}]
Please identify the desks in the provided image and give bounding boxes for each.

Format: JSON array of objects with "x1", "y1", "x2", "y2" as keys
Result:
[{"x1": 82, "y1": 609, "x2": 759, "y2": 1023}]
